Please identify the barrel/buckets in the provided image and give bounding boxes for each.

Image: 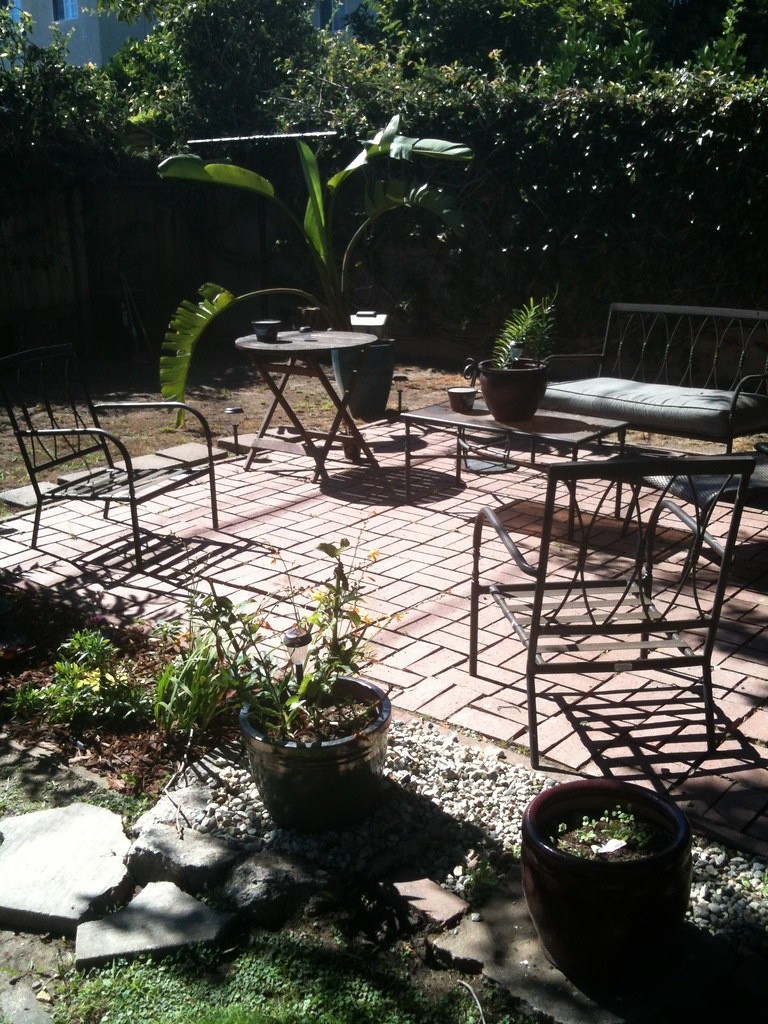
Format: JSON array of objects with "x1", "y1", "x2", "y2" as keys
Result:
[
  {"x1": 251, "y1": 319, "x2": 282, "y2": 342},
  {"x1": 445, "y1": 386, "x2": 478, "y2": 411},
  {"x1": 340, "y1": 432, "x2": 365, "y2": 459}
]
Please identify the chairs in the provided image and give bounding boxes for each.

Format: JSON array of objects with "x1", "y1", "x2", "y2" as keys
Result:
[
  {"x1": 470, "y1": 456, "x2": 756, "y2": 773},
  {"x1": 0, "y1": 341, "x2": 218, "y2": 570}
]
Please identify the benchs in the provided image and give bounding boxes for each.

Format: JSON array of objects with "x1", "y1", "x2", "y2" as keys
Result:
[
  {"x1": 538, "y1": 302, "x2": 768, "y2": 455},
  {"x1": 618, "y1": 441, "x2": 768, "y2": 575}
]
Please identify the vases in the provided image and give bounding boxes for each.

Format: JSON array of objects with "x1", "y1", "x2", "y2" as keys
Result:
[
  {"x1": 251, "y1": 319, "x2": 282, "y2": 342},
  {"x1": 477, "y1": 285, "x2": 559, "y2": 425}
]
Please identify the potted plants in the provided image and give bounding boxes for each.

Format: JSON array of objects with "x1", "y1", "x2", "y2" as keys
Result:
[
  {"x1": 520, "y1": 779, "x2": 693, "y2": 989},
  {"x1": 156, "y1": 114, "x2": 475, "y2": 431},
  {"x1": 447, "y1": 386, "x2": 478, "y2": 412},
  {"x1": 190, "y1": 540, "x2": 404, "y2": 844}
]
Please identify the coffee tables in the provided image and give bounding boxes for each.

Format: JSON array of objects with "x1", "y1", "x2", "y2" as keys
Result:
[{"x1": 400, "y1": 397, "x2": 629, "y2": 542}]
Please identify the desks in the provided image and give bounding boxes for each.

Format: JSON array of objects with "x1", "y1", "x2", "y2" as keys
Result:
[{"x1": 235, "y1": 330, "x2": 379, "y2": 485}]
